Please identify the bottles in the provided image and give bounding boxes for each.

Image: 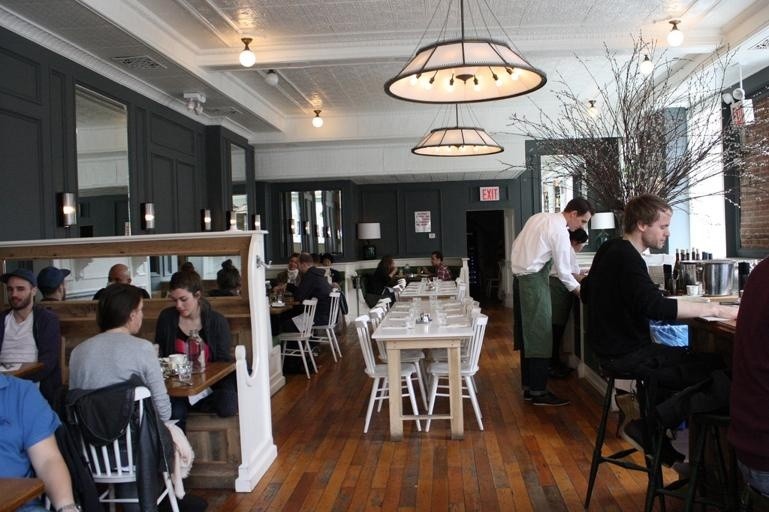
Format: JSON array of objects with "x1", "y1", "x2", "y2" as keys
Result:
[
  {"x1": 673, "y1": 248, "x2": 713, "y2": 280},
  {"x1": 187, "y1": 329, "x2": 205, "y2": 373}
]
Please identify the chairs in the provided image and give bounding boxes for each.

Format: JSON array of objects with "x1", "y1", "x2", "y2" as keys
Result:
[{"x1": 276, "y1": 277, "x2": 490, "y2": 434}]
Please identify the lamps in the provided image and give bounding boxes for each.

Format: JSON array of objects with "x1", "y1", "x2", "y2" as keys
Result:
[
  {"x1": 183, "y1": 92, "x2": 206, "y2": 115},
  {"x1": 357, "y1": 222, "x2": 382, "y2": 258},
  {"x1": 590, "y1": 212, "x2": 617, "y2": 245},
  {"x1": 587, "y1": 19, "x2": 684, "y2": 118},
  {"x1": 56, "y1": 191, "x2": 341, "y2": 240},
  {"x1": 722, "y1": 65, "x2": 755, "y2": 127},
  {"x1": 238, "y1": 36, "x2": 325, "y2": 129},
  {"x1": 383, "y1": 1, "x2": 548, "y2": 104},
  {"x1": 411, "y1": 103, "x2": 504, "y2": 157}
]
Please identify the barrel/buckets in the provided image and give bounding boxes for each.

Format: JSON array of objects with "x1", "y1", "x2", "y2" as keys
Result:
[
  {"x1": 703, "y1": 259, "x2": 734, "y2": 296},
  {"x1": 680, "y1": 259, "x2": 704, "y2": 290}
]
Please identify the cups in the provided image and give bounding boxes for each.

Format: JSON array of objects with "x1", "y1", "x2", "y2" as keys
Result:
[
  {"x1": 686, "y1": 285, "x2": 699, "y2": 296},
  {"x1": 169, "y1": 354, "x2": 193, "y2": 380}
]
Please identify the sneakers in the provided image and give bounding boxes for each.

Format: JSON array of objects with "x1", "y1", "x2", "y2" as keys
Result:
[
  {"x1": 619, "y1": 419, "x2": 686, "y2": 468},
  {"x1": 524, "y1": 391, "x2": 571, "y2": 407}
]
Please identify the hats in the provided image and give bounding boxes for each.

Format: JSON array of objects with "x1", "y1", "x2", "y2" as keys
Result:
[
  {"x1": 0, "y1": 268, "x2": 37, "y2": 286},
  {"x1": 37, "y1": 267, "x2": 72, "y2": 292}
]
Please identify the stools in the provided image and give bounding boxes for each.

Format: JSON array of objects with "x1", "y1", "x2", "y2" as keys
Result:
[{"x1": 584, "y1": 360, "x2": 769, "y2": 512}]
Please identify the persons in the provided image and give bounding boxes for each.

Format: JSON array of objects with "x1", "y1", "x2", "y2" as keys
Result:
[
  {"x1": 368, "y1": 257, "x2": 397, "y2": 295},
  {"x1": 728, "y1": 256, "x2": 769, "y2": 497},
  {"x1": 511, "y1": 197, "x2": 594, "y2": 405},
  {"x1": 1, "y1": 259, "x2": 242, "y2": 512},
  {"x1": 547, "y1": 228, "x2": 589, "y2": 378},
  {"x1": 265, "y1": 253, "x2": 341, "y2": 373},
  {"x1": 423, "y1": 250, "x2": 451, "y2": 281},
  {"x1": 581, "y1": 194, "x2": 739, "y2": 469}
]
